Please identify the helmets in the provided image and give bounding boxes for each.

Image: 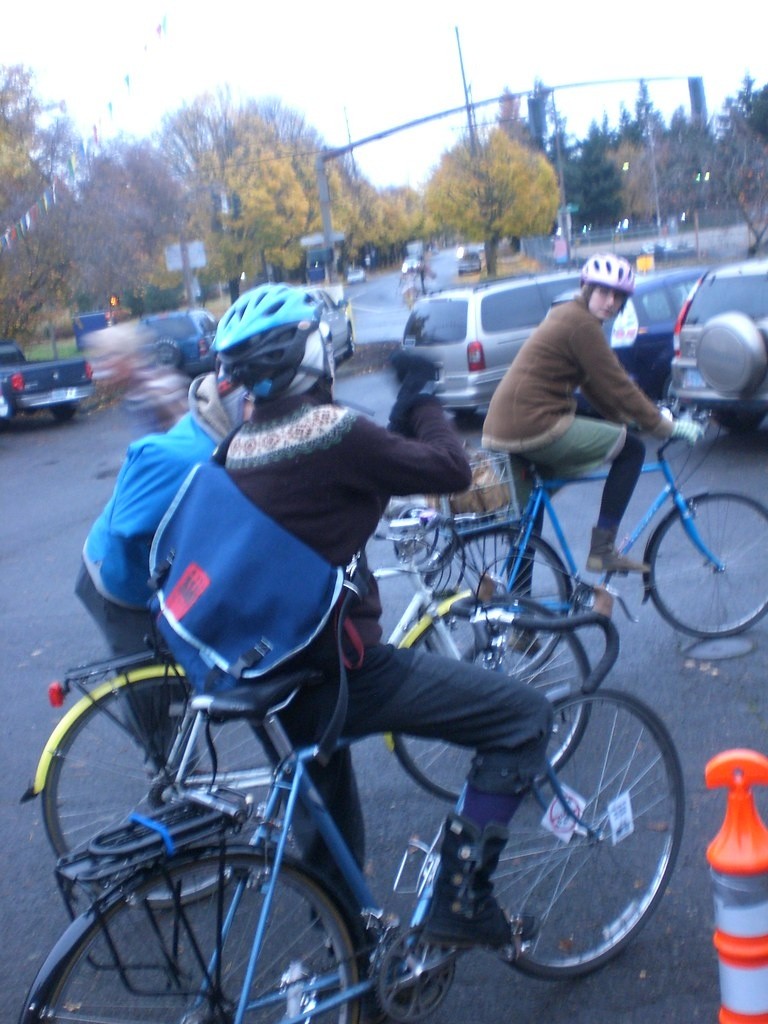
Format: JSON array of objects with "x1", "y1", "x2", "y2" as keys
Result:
[
  {"x1": 210, "y1": 283, "x2": 325, "y2": 401},
  {"x1": 579, "y1": 253, "x2": 634, "y2": 294}
]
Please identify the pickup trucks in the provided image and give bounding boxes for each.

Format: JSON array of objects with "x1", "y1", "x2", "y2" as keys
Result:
[{"x1": 0, "y1": 338, "x2": 96, "y2": 428}]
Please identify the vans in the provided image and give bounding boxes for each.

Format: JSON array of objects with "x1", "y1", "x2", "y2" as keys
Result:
[{"x1": 402, "y1": 268, "x2": 586, "y2": 421}]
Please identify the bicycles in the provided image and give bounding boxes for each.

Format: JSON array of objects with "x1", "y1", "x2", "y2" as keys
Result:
[
  {"x1": 419, "y1": 398, "x2": 768, "y2": 682},
  {"x1": 19, "y1": 441, "x2": 593, "y2": 909},
  {"x1": 12, "y1": 534, "x2": 685, "y2": 1024}
]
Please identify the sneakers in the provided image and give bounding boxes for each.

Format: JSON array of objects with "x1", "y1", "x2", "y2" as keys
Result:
[{"x1": 507, "y1": 611, "x2": 541, "y2": 658}]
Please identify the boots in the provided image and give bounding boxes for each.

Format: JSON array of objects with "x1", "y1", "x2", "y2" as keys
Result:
[
  {"x1": 328, "y1": 925, "x2": 454, "y2": 1024},
  {"x1": 419, "y1": 811, "x2": 541, "y2": 949}
]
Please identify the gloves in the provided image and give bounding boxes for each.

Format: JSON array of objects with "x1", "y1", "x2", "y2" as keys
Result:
[
  {"x1": 389, "y1": 310, "x2": 447, "y2": 403},
  {"x1": 671, "y1": 420, "x2": 705, "y2": 447}
]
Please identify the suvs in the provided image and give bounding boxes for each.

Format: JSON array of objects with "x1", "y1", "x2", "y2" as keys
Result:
[
  {"x1": 669, "y1": 257, "x2": 768, "y2": 433},
  {"x1": 137, "y1": 312, "x2": 217, "y2": 381},
  {"x1": 546, "y1": 265, "x2": 710, "y2": 431}
]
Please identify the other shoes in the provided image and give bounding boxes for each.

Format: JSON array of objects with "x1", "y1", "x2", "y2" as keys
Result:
[{"x1": 586, "y1": 527, "x2": 650, "y2": 573}]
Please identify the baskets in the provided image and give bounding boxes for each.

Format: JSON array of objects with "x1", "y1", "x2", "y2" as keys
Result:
[{"x1": 395, "y1": 449, "x2": 521, "y2": 539}]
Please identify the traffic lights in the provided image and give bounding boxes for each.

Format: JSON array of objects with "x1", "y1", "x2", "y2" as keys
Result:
[{"x1": 110, "y1": 295, "x2": 118, "y2": 305}]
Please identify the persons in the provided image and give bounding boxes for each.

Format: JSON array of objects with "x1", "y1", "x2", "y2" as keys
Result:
[
  {"x1": 480, "y1": 252, "x2": 705, "y2": 657},
  {"x1": 74, "y1": 365, "x2": 256, "y2": 782},
  {"x1": 85, "y1": 324, "x2": 190, "y2": 439},
  {"x1": 213, "y1": 283, "x2": 555, "y2": 1002}
]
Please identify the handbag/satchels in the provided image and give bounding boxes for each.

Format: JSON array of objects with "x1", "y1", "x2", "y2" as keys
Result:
[{"x1": 147, "y1": 420, "x2": 363, "y2": 766}]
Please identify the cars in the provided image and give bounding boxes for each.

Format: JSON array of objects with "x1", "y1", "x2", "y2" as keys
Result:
[
  {"x1": 347, "y1": 268, "x2": 365, "y2": 283},
  {"x1": 459, "y1": 253, "x2": 482, "y2": 274}
]
